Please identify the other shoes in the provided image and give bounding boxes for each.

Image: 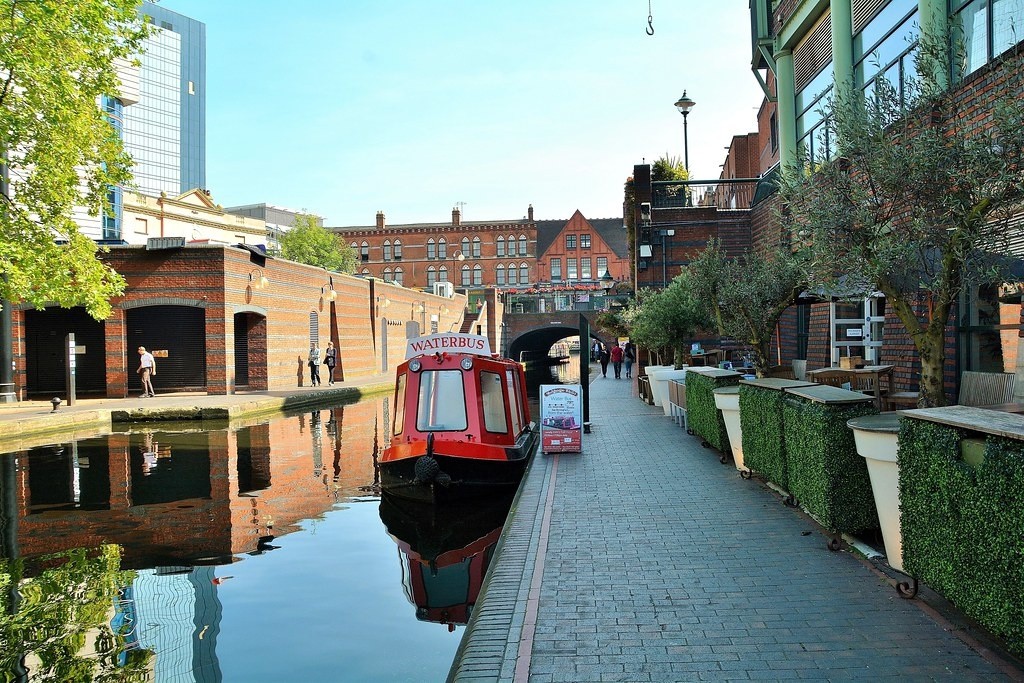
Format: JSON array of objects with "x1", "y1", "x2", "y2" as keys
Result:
[
  {"x1": 138, "y1": 394, "x2": 155, "y2": 397},
  {"x1": 604, "y1": 374, "x2": 606, "y2": 377},
  {"x1": 329, "y1": 382, "x2": 334, "y2": 386},
  {"x1": 311, "y1": 383, "x2": 315, "y2": 387},
  {"x1": 615, "y1": 376, "x2": 620, "y2": 379},
  {"x1": 626, "y1": 372, "x2": 631, "y2": 378},
  {"x1": 318, "y1": 382, "x2": 321, "y2": 387}
]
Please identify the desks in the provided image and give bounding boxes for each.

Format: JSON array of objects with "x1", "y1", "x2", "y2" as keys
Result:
[
  {"x1": 691, "y1": 351, "x2": 718, "y2": 369},
  {"x1": 808, "y1": 366, "x2": 895, "y2": 412},
  {"x1": 736, "y1": 367, "x2": 757, "y2": 375}
]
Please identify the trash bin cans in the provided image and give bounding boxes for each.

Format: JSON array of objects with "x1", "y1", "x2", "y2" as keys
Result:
[{"x1": 516, "y1": 303, "x2": 523, "y2": 313}]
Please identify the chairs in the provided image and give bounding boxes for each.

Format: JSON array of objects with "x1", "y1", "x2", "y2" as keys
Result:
[{"x1": 681, "y1": 350, "x2": 921, "y2": 412}]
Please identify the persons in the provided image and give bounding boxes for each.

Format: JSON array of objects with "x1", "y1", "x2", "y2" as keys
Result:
[
  {"x1": 475, "y1": 298, "x2": 482, "y2": 313},
  {"x1": 591, "y1": 340, "x2": 635, "y2": 379},
  {"x1": 136, "y1": 346, "x2": 157, "y2": 398},
  {"x1": 326, "y1": 341, "x2": 337, "y2": 386},
  {"x1": 308, "y1": 343, "x2": 321, "y2": 387}
]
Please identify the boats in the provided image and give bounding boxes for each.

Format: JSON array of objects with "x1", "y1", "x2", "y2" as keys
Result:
[
  {"x1": 569, "y1": 340, "x2": 580, "y2": 351},
  {"x1": 375, "y1": 332, "x2": 536, "y2": 501},
  {"x1": 378, "y1": 491, "x2": 514, "y2": 632}
]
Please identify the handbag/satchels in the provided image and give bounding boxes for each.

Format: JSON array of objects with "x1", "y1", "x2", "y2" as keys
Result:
[{"x1": 323, "y1": 358, "x2": 327, "y2": 364}]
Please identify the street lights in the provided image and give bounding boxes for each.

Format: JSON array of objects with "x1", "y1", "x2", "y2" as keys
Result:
[{"x1": 674, "y1": 89, "x2": 695, "y2": 207}]
